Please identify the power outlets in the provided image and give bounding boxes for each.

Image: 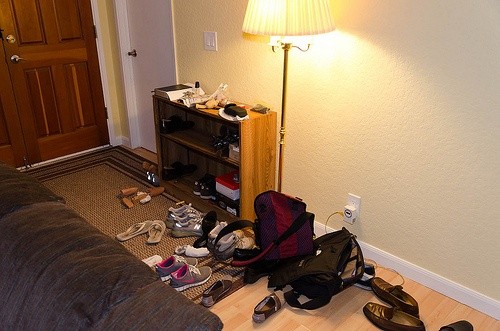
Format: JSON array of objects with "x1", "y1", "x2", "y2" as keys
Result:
[{"x1": 345, "y1": 193, "x2": 360, "y2": 221}]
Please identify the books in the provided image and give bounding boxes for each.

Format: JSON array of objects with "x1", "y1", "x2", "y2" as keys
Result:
[{"x1": 155, "y1": 83, "x2": 193, "y2": 102}]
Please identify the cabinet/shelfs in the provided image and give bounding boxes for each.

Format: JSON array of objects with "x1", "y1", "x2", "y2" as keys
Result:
[{"x1": 151, "y1": 85, "x2": 276, "y2": 221}]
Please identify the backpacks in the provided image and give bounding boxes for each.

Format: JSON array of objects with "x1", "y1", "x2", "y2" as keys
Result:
[{"x1": 268, "y1": 227, "x2": 365, "y2": 310}]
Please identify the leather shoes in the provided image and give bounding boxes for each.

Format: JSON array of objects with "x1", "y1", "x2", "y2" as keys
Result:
[
  {"x1": 371, "y1": 277, "x2": 419, "y2": 316},
  {"x1": 363, "y1": 302, "x2": 426, "y2": 331}
]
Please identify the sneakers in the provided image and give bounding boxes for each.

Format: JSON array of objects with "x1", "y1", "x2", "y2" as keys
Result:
[
  {"x1": 154, "y1": 255, "x2": 198, "y2": 281},
  {"x1": 169, "y1": 264, "x2": 212, "y2": 291},
  {"x1": 212, "y1": 232, "x2": 254, "y2": 261},
  {"x1": 208, "y1": 221, "x2": 244, "y2": 249},
  {"x1": 166, "y1": 203, "x2": 203, "y2": 228},
  {"x1": 171, "y1": 214, "x2": 203, "y2": 238},
  {"x1": 194, "y1": 174, "x2": 216, "y2": 199}
]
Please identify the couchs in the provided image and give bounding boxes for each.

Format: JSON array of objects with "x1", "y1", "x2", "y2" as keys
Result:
[{"x1": 0, "y1": 169, "x2": 223, "y2": 331}]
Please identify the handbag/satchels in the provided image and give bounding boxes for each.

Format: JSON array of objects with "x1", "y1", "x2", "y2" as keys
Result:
[{"x1": 254, "y1": 189, "x2": 315, "y2": 260}]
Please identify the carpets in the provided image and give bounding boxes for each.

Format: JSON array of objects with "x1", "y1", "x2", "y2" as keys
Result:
[{"x1": 40, "y1": 146, "x2": 247, "y2": 309}]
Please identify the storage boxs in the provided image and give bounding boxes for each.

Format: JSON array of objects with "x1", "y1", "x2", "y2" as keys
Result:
[{"x1": 215, "y1": 171, "x2": 240, "y2": 217}]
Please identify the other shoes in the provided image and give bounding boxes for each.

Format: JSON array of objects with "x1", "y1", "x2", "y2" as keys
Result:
[
  {"x1": 201, "y1": 275, "x2": 232, "y2": 307},
  {"x1": 252, "y1": 290, "x2": 287, "y2": 323},
  {"x1": 162, "y1": 161, "x2": 198, "y2": 182},
  {"x1": 160, "y1": 115, "x2": 195, "y2": 133},
  {"x1": 439, "y1": 320, "x2": 473, "y2": 331}
]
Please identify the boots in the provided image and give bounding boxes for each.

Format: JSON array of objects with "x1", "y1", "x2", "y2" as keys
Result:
[{"x1": 193, "y1": 210, "x2": 217, "y2": 248}]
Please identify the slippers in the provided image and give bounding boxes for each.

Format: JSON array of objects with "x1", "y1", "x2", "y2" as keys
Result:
[
  {"x1": 146, "y1": 220, "x2": 165, "y2": 243},
  {"x1": 116, "y1": 220, "x2": 153, "y2": 241}
]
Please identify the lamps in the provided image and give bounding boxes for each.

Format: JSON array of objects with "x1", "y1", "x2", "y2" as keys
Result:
[{"x1": 242, "y1": 0, "x2": 334, "y2": 193}]
